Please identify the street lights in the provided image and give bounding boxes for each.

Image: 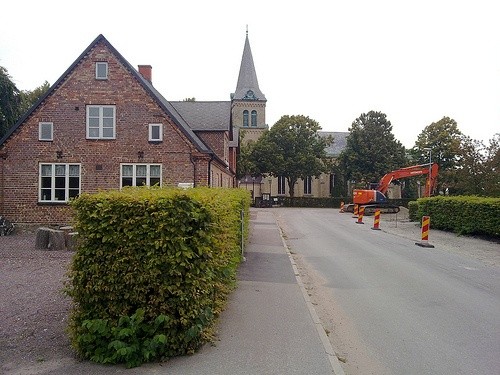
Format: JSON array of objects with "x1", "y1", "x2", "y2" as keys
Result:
[{"x1": 423, "y1": 148, "x2": 432, "y2": 197}]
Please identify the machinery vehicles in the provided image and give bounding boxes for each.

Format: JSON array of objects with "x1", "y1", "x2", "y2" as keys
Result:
[{"x1": 342, "y1": 162, "x2": 439, "y2": 216}]
